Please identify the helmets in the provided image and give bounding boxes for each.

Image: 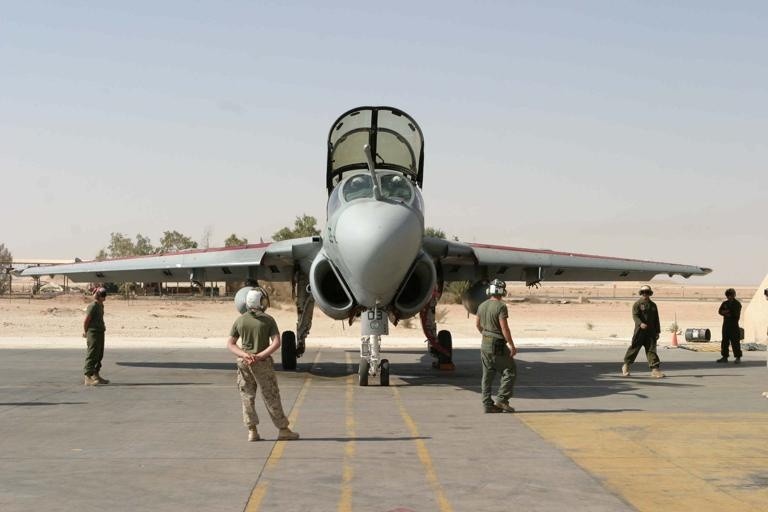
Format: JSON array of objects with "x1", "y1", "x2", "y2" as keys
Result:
[
  {"x1": 726, "y1": 289, "x2": 736, "y2": 298},
  {"x1": 639, "y1": 285, "x2": 652, "y2": 296},
  {"x1": 92, "y1": 287, "x2": 106, "y2": 299},
  {"x1": 486, "y1": 278, "x2": 506, "y2": 296},
  {"x1": 246, "y1": 287, "x2": 270, "y2": 312}
]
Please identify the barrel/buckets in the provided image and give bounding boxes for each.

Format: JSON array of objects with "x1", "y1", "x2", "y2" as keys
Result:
[{"x1": 685, "y1": 328, "x2": 711, "y2": 342}]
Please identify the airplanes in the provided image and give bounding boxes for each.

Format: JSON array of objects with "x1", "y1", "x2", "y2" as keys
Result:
[{"x1": 6, "y1": 104, "x2": 714, "y2": 393}]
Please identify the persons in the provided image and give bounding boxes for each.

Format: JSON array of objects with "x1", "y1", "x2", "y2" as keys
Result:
[
  {"x1": 622, "y1": 285, "x2": 665, "y2": 378},
  {"x1": 82, "y1": 287, "x2": 108, "y2": 384},
  {"x1": 475, "y1": 278, "x2": 517, "y2": 413},
  {"x1": 717, "y1": 288, "x2": 742, "y2": 363},
  {"x1": 227, "y1": 286, "x2": 299, "y2": 441}
]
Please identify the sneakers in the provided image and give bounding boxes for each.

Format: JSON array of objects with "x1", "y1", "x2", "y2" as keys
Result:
[
  {"x1": 487, "y1": 408, "x2": 502, "y2": 412},
  {"x1": 494, "y1": 401, "x2": 514, "y2": 411},
  {"x1": 278, "y1": 432, "x2": 299, "y2": 440},
  {"x1": 248, "y1": 430, "x2": 259, "y2": 441}
]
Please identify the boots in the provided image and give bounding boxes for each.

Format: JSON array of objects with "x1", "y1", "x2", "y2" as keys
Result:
[
  {"x1": 652, "y1": 368, "x2": 664, "y2": 378},
  {"x1": 717, "y1": 357, "x2": 727, "y2": 362},
  {"x1": 622, "y1": 364, "x2": 630, "y2": 375},
  {"x1": 94, "y1": 375, "x2": 108, "y2": 384},
  {"x1": 85, "y1": 376, "x2": 99, "y2": 385},
  {"x1": 735, "y1": 357, "x2": 739, "y2": 363}
]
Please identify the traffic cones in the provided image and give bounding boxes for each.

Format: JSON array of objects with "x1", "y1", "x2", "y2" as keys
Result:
[{"x1": 669, "y1": 330, "x2": 679, "y2": 347}]
